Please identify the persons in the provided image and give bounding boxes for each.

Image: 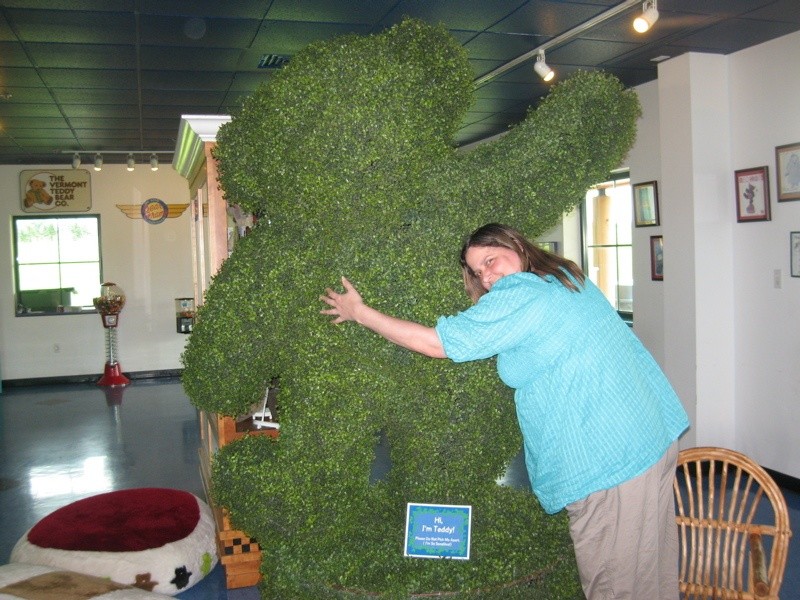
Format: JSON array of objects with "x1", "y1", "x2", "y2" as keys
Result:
[{"x1": 319, "y1": 223, "x2": 689, "y2": 600}]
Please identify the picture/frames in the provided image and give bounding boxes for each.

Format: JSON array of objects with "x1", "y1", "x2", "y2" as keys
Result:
[
  {"x1": 633, "y1": 181, "x2": 660, "y2": 228},
  {"x1": 790, "y1": 231, "x2": 800, "y2": 277},
  {"x1": 734, "y1": 165, "x2": 771, "y2": 223},
  {"x1": 775, "y1": 142, "x2": 800, "y2": 202},
  {"x1": 650, "y1": 235, "x2": 663, "y2": 281}
]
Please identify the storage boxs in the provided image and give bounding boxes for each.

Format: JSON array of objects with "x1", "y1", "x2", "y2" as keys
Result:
[{"x1": 19, "y1": 287, "x2": 73, "y2": 313}]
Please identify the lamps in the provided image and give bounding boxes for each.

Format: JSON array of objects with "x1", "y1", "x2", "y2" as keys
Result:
[
  {"x1": 533, "y1": 51, "x2": 554, "y2": 81},
  {"x1": 633, "y1": 0, "x2": 659, "y2": 32},
  {"x1": 150, "y1": 153, "x2": 159, "y2": 171},
  {"x1": 93, "y1": 153, "x2": 103, "y2": 172},
  {"x1": 127, "y1": 153, "x2": 134, "y2": 171},
  {"x1": 71, "y1": 152, "x2": 81, "y2": 169}
]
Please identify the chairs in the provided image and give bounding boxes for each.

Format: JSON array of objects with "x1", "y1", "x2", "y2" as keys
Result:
[{"x1": 673, "y1": 446, "x2": 792, "y2": 600}]
